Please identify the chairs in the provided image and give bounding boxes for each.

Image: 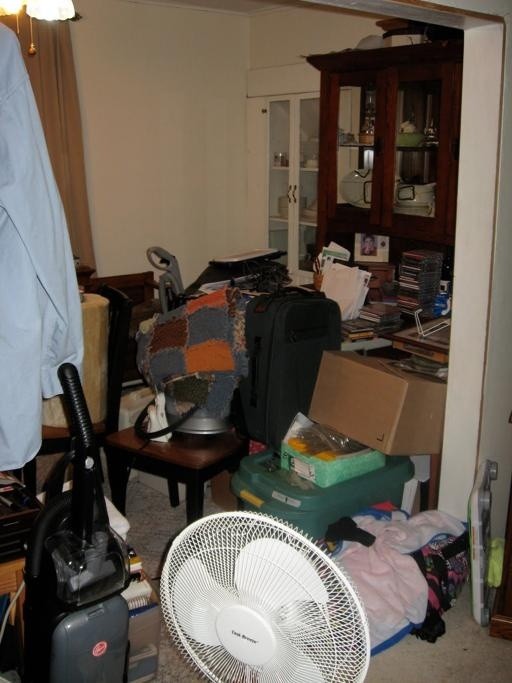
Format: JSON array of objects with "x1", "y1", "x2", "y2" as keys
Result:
[{"x1": 12, "y1": 283, "x2": 136, "y2": 540}]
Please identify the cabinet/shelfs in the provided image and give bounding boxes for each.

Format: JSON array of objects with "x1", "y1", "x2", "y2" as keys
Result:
[
  {"x1": 246, "y1": 63, "x2": 361, "y2": 288},
  {"x1": 306, "y1": 42, "x2": 464, "y2": 363}
]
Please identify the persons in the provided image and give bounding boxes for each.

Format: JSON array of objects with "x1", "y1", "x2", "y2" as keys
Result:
[{"x1": 361, "y1": 235, "x2": 376, "y2": 256}]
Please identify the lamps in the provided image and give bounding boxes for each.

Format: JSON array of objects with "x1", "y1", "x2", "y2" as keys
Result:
[{"x1": 0, "y1": 0, "x2": 82, "y2": 57}]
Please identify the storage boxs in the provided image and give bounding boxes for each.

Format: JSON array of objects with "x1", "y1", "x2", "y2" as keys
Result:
[
  {"x1": 306, "y1": 349, "x2": 448, "y2": 458},
  {"x1": 123, "y1": 570, "x2": 159, "y2": 683},
  {"x1": 230, "y1": 449, "x2": 416, "y2": 544}
]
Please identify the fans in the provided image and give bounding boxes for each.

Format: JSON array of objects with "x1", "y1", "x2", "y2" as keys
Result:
[{"x1": 157, "y1": 509, "x2": 371, "y2": 683}]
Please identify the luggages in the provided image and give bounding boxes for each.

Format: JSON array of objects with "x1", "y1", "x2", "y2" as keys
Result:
[
  {"x1": 24, "y1": 595, "x2": 129, "y2": 683},
  {"x1": 227, "y1": 282, "x2": 342, "y2": 454}
]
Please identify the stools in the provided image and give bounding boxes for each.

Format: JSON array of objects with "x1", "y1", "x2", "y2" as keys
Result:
[{"x1": 104, "y1": 423, "x2": 243, "y2": 528}]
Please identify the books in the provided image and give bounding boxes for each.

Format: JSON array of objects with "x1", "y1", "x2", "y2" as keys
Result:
[{"x1": 320, "y1": 248, "x2": 452, "y2": 382}]
